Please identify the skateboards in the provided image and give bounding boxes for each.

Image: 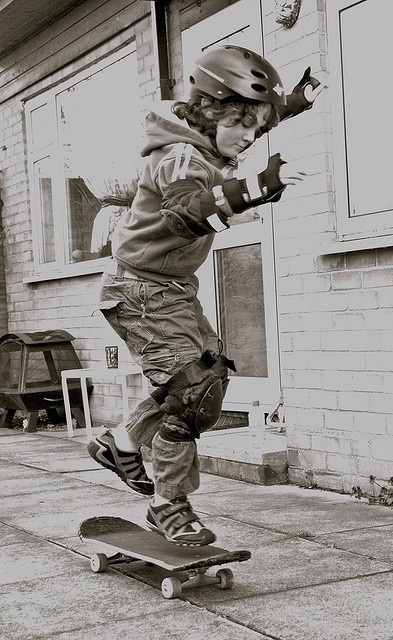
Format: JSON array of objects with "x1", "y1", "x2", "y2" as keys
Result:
[{"x1": 77, "y1": 516, "x2": 251, "y2": 599}]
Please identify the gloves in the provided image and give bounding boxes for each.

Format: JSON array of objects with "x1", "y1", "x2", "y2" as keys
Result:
[
  {"x1": 221, "y1": 152, "x2": 285, "y2": 219},
  {"x1": 271, "y1": 66, "x2": 318, "y2": 124}
]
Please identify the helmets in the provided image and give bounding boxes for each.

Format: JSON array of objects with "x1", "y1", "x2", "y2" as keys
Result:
[{"x1": 189, "y1": 43, "x2": 286, "y2": 107}]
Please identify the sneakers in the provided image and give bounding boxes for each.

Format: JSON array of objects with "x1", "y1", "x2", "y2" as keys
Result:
[
  {"x1": 86, "y1": 430, "x2": 153, "y2": 496},
  {"x1": 145, "y1": 495, "x2": 216, "y2": 548}
]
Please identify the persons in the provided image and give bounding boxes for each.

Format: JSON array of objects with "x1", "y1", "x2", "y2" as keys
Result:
[{"x1": 87, "y1": 44, "x2": 329, "y2": 548}]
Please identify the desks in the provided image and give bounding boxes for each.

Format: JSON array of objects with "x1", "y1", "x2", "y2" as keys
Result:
[{"x1": 61, "y1": 364, "x2": 148, "y2": 445}]
